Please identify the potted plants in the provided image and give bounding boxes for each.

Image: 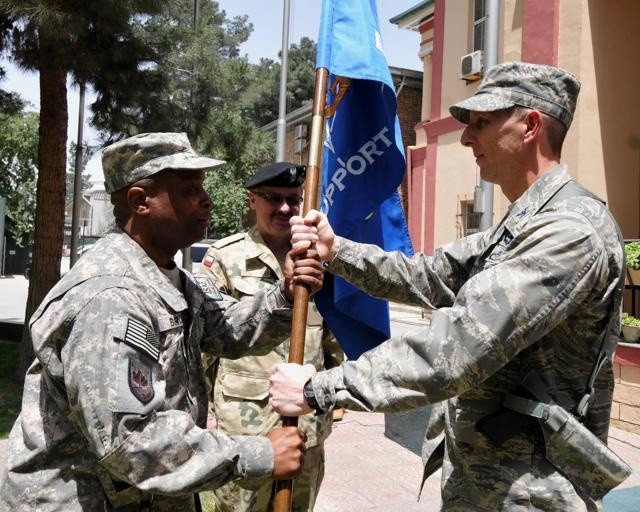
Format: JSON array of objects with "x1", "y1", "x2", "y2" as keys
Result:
[{"x1": 618, "y1": 241, "x2": 640, "y2": 343}]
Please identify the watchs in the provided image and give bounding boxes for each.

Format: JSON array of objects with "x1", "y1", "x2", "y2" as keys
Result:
[{"x1": 302, "y1": 374, "x2": 328, "y2": 418}]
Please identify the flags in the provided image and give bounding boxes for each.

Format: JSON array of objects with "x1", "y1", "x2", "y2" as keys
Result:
[{"x1": 303, "y1": 1, "x2": 414, "y2": 362}]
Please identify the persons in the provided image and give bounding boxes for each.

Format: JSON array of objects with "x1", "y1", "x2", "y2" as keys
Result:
[
  {"x1": 195, "y1": 162, "x2": 346, "y2": 510},
  {"x1": 0, "y1": 126, "x2": 326, "y2": 510},
  {"x1": 265, "y1": 59, "x2": 634, "y2": 512}
]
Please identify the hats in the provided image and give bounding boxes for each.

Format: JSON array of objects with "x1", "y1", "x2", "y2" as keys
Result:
[
  {"x1": 99, "y1": 128, "x2": 229, "y2": 195},
  {"x1": 243, "y1": 161, "x2": 307, "y2": 189},
  {"x1": 448, "y1": 61, "x2": 581, "y2": 131}
]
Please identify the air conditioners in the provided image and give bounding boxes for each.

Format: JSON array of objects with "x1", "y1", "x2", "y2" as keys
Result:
[
  {"x1": 292, "y1": 124, "x2": 308, "y2": 154},
  {"x1": 459, "y1": 50, "x2": 485, "y2": 81}
]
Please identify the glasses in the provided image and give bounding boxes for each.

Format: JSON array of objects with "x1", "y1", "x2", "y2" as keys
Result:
[{"x1": 255, "y1": 192, "x2": 305, "y2": 207}]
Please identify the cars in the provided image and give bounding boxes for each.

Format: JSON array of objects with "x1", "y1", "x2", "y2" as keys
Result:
[
  {"x1": 173, "y1": 243, "x2": 211, "y2": 275},
  {"x1": 60, "y1": 243, "x2": 96, "y2": 279}
]
